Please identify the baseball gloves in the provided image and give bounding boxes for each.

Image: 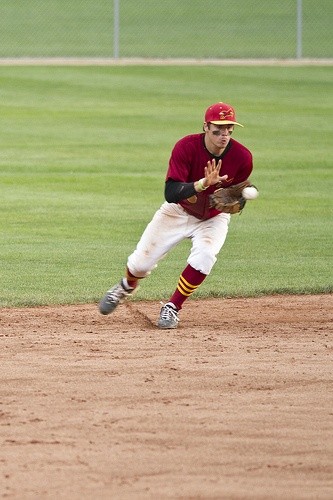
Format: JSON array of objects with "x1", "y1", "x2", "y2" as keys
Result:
[{"x1": 209, "y1": 181, "x2": 250, "y2": 216}]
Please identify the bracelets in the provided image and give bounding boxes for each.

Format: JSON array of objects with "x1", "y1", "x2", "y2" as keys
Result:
[{"x1": 198, "y1": 179, "x2": 209, "y2": 191}]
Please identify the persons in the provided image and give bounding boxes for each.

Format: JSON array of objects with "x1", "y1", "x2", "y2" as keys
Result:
[{"x1": 99, "y1": 102, "x2": 256, "y2": 330}]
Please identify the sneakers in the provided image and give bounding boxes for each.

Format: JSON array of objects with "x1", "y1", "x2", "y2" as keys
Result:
[
  {"x1": 158, "y1": 300, "x2": 180, "y2": 328},
  {"x1": 99, "y1": 277, "x2": 134, "y2": 315}
]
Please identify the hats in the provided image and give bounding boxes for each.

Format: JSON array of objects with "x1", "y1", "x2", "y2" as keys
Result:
[{"x1": 205, "y1": 102, "x2": 244, "y2": 127}]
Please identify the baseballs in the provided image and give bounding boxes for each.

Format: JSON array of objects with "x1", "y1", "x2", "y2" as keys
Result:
[{"x1": 242, "y1": 187, "x2": 257, "y2": 199}]
